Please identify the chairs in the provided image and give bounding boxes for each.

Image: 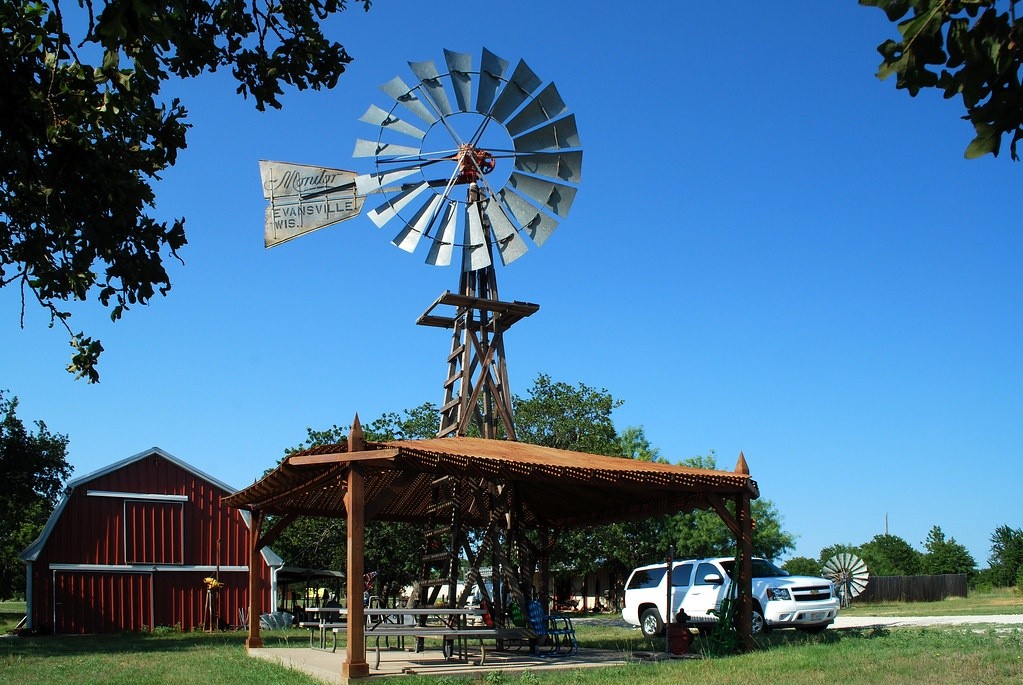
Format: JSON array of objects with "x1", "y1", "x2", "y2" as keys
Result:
[{"x1": 527, "y1": 601, "x2": 578, "y2": 657}]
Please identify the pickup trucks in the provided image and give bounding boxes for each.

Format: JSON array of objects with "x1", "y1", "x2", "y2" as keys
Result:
[{"x1": 622, "y1": 555, "x2": 842, "y2": 636}]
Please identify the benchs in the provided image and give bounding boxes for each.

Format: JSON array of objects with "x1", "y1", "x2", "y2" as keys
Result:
[{"x1": 301, "y1": 606, "x2": 540, "y2": 665}]
